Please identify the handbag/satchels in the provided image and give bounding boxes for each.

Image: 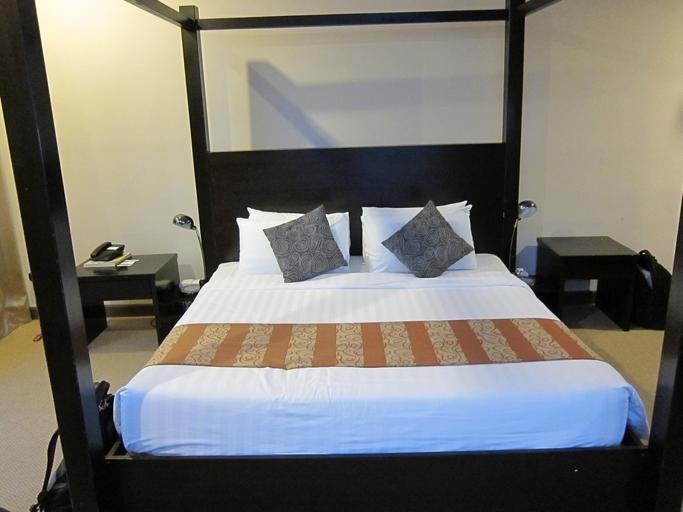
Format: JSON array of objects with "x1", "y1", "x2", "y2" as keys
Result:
[
  {"x1": 634, "y1": 249, "x2": 671, "y2": 330},
  {"x1": 28, "y1": 380, "x2": 114, "y2": 512}
]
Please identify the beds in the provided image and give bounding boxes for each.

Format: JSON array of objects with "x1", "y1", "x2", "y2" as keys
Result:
[{"x1": 65, "y1": 252, "x2": 682, "y2": 511}]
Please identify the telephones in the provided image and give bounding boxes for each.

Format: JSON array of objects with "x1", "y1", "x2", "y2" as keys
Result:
[{"x1": 90, "y1": 242, "x2": 125, "y2": 261}]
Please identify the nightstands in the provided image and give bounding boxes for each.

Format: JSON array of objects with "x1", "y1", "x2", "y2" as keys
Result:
[
  {"x1": 535, "y1": 234, "x2": 641, "y2": 336},
  {"x1": 74, "y1": 249, "x2": 181, "y2": 347}
]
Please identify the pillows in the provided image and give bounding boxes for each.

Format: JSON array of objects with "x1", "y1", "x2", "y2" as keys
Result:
[
  {"x1": 357, "y1": 205, "x2": 476, "y2": 272},
  {"x1": 235, "y1": 216, "x2": 349, "y2": 275},
  {"x1": 264, "y1": 203, "x2": 347, "y2": 283},
  {"x1": 380, "y1": 200, "x2": 472, "y2": 280},
  {"x1": 247, "y1": 207, "x2": 349, "y2": 225},
  {"x1": 360, "y1": 199, "x2": 470, "y2": 221}
]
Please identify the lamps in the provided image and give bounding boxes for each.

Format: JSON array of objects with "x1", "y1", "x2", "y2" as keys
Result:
[
  {"x1": 509, "y1": 198, "x2": 536, "y2": 273},
  {"x1": 174, "y1": 213, "x2": 203, "y2": 250}
]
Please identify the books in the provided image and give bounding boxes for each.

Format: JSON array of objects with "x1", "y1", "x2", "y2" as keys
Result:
[{"x1": 84, "y1": 253, "x2": 132, "y2": 268}]
